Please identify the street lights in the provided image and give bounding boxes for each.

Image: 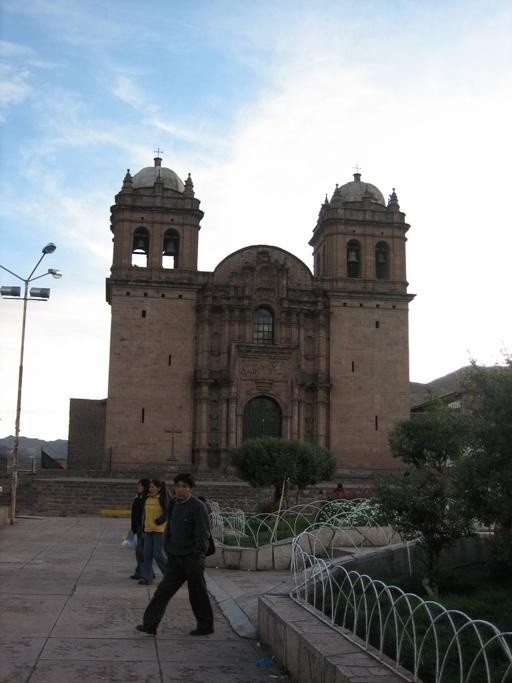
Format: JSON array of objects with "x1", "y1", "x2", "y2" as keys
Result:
[{"x1": 2, "y1": 242, "x2": 64, "y2": 523}]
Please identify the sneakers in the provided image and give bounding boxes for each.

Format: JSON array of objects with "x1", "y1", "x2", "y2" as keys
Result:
[
  {"x1": 130, "y1": 575, "x2": 156, "y2": 585},
  {"x1": 190, "y1": 629, "x2": 213, "y2": 635},
  {"x1": 136, "y1": 625, "x2": 157, "y2": 635}
]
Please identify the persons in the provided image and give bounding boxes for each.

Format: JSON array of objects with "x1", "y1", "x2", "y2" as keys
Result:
[
  {"x1": 333, "y1": 483, "x2": 346, "y2": 498},
  {"x1": 129, "y1": 477, "x2": 151, "y2": 579},
  {"x1": 137, "y1": 473, "x2": 214, "y2": 635},
  {"x1": 138, "y1": 478, "x2": 173, "y2": 585}
]
